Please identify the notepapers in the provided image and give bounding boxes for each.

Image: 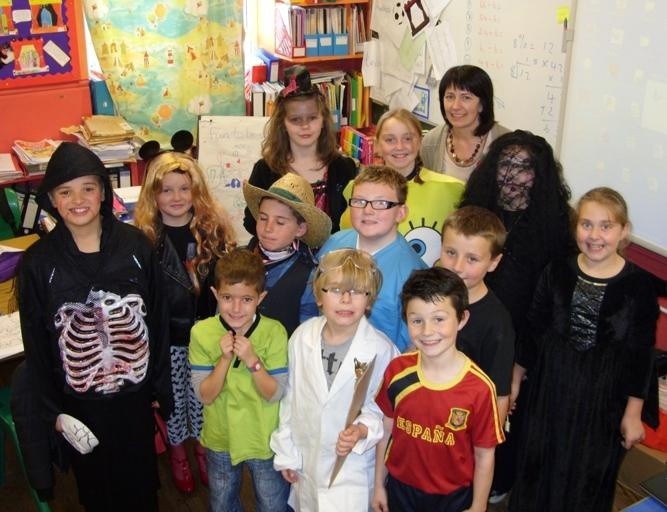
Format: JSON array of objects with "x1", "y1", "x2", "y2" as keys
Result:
[{"x1": 556, "y1": 7, "x2": 570, "y2": 25}]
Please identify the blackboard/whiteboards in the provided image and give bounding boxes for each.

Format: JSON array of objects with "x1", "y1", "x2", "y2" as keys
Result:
[
  {"x1": 366, "y1": 0, "x2": 572, "y2": 198},
  {"x1": 531, "y1": 0, "x2": 667, "y2": 257}
]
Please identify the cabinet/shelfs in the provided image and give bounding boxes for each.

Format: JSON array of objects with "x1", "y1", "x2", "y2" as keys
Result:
[
  {"x1": 257, "y1": 0, "x2": 372, "y2": 133},
  {"x1": 0, "y1": 0, "x2": 92, "y2": 153}
]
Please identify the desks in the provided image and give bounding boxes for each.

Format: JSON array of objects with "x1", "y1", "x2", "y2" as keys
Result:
[{"x1": 0, "y1": 135, "x2": 148, "y2": 186}]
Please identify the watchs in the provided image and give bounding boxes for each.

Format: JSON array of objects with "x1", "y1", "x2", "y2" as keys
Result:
[{"x1": 246, "y1": 356, "x2": 263, "y2": 373}]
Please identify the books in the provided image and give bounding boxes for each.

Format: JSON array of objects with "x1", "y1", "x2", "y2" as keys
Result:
[
  {"x1": 251, "y1": 47, "x2": 370, "y2": 129},
  {"x1": 0, "y1": 152, "x2": 25, "y2": 180},
  {"x1": 62, "y1": 114, "x2": 135, "y2": 162},
  {"x1": 10, "y1": 139, "x2": 64, "y2": 177}
]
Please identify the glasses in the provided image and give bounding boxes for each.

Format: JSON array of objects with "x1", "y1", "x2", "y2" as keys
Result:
[
  {"x1": 348, "y1": 197, "x2": 406, "y2": 211},
  {"x1": 321, "y1": 287, "x2": 373, "y2": 297}
]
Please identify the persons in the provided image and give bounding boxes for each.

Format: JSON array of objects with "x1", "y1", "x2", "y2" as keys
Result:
[
  {"x1": 240, "y1": 173, "x2": 334, "y2": 340},
  {"x1": 298, "y1": 164, "x2": 430, "y2": 355},
  {"x1": 439, "y1": 203, "x2": 516, "y2": 432},
  {"x1": 135, "y1": 131, "x2": 238, "y2": 492},
  {"x1": 189, "y1": 247, "x2": 291, "y2": 512},
  {"x1": 242, "y1": 64, "x2": 360, "y2": 250},
  {"x1": 510, "y1": 184, "x2": 660, "y2": 512},
  {"x1": 269, "y1": 247, "x2": 401, "y2": 512},
  {"x1": 418, "y1": 68, "x2": 511, "y2": 184},
  {"x1": 337, "y1": 107, "x2": 468, "y2": 270},
  {"x1": 13, "y1": 143, "x2": 176, "y2": 511},
  {"x1": 452, "y1": 129, "x2": 575, "y2": 505},
  {"x1": 370, "y1": 265, "x2": 508, "y2": 512}
]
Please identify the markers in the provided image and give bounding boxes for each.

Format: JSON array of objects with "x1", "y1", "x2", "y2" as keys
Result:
[{"x1": 561, "y1": 17, "x2": 567, "y2": 52}]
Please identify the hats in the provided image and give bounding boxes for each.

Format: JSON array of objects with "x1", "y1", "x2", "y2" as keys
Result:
[
  {"x1": 242, "y1": 174, "x2": 334, "y2": 250},
  {"x1": 278, "y1": 64, "x2": 321, "y2": 98}
]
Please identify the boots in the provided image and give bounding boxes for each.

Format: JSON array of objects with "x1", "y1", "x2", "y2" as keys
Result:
[
  {"x1": 196, "y1": 444, "x2": 210, "y2": 490},
  {"x1": 170, "y1": 448, "x2": 196, "y2": 496}
]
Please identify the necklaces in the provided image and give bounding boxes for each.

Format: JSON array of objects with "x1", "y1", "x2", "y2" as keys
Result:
[{"x1": 447, "y1": 128, "x2": 484, "y2": 166}]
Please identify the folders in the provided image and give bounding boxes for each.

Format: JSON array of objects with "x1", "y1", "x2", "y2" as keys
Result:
[
  {"x1": 108, "y1": 169, "x2": 119, "y2": 188},
  {"x1": 117, "y1": 168, "x2": 131, "y2": 186},
  {"x1": 18, "y1": 183, "x2": 41, "y2": 235},
  {"x1": 328, "y1": 354, "x2": 378, "y2": 491},
  {"x1": 351, "y1": 68, "x2": 366, "y2": 129},
  {"x1": 263, "y1": 81, "x2": 277, "y2": 116},
  {"x1": 362, "y1": 77, "x2": 371, "y2": 127},
  {"x1": 346, "y1": 74, "x2": 357, "y2": 126},
  {"x1": 11, "y1": 182, "x2": 26, "y2": 210},
  {"x1": 89, "y1": 70, "x2": 114, "y2": 115},
  {"x1": 252, "y1": 82, "x2": 264, "y2": 116}
]
